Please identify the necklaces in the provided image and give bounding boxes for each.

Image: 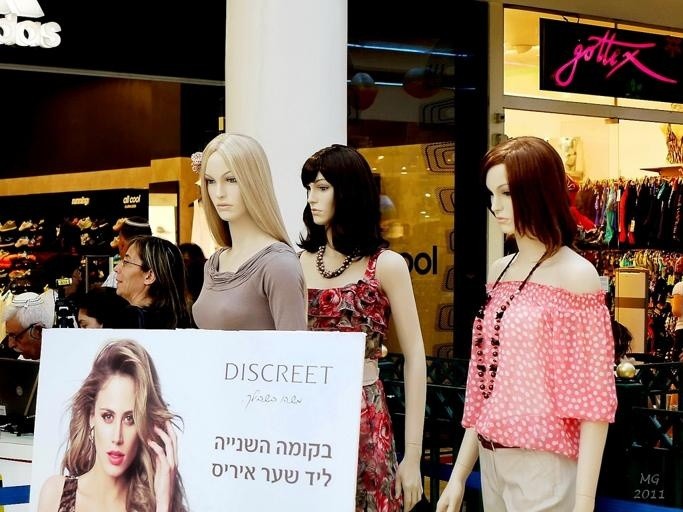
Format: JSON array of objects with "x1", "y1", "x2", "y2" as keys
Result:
[
  {"x1": 473, "y1": 245, "x2": 549, "y2": 399},
  {"x1": 315, "y1": 244, "x2": 360, "y2": 281}
]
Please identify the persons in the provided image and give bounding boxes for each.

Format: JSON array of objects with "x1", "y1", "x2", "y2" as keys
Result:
[
  {"x1": 100, "y1": 217, "x2": 152, "y2": 288},
  {"x1": 78, "y1": 286, "x2": 144, "y2": 328},
  {"x1": 191, "y1": 133, "x2": 307, "y2": 331},
  {"x1": 113, "y1": 236, "x2": 198, "y2": 329},
  {"x1": 433, "y1": 137, "x2": 619, "y2": 512},
  {"x1": 5, "y1": 292, "x2": 54, "y2": 362},
  {"x1": 668, "y1": 258, "x2": 683, "y2": 361},
  {"x1": 293, "y1": 143, "x2": 429, "y2": 512},
  {"x1": 36, "y1": 340, "x2": 190, "y2": 512},
  {"x1": 176, "y1": 241, "x2": 203, "y2": 316},
  {"x1": 40, "y1": 254, "x2": 83, "y2": 328}
]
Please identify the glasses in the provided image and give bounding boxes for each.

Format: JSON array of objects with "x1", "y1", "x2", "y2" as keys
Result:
[
  {"x1": 8, "y1": 323, "x2": 36, "y2": 341},
  {"x1": 119, "y1": 258, "x2": 145, "y2": 270}
]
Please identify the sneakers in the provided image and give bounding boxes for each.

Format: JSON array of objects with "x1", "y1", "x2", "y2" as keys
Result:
[{"x1": 0, "y1": 216, "x2": 129, "y2": 301}]
